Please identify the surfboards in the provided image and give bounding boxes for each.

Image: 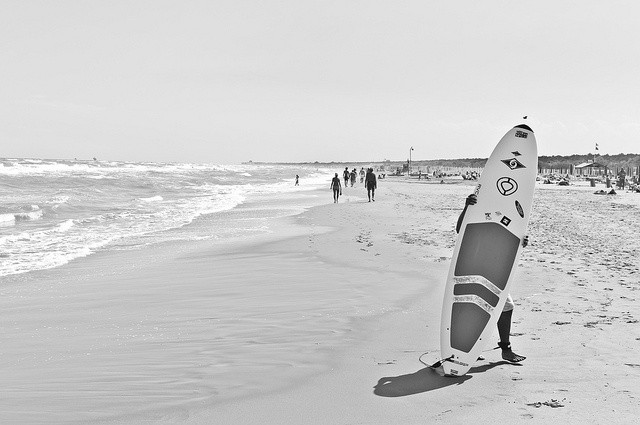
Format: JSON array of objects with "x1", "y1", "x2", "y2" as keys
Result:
[{"x1": 419, "y1": 124, "x2": 538, "y2": 377}]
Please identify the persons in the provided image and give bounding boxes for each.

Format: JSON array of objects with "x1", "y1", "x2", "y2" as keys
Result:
[
  {"x1": 456, "y1": 194, "x2": 529, "y2": 362},
  {"x1": 329, "y1": 173, "x2": 342, "y2": 204},
  {"x1": 626, "y1": 173, "x2": 639, "y2": 194},
  {"x1": 343, "y1": 166, "x2": 350, "y2": 187},
  {"x1": 618, "y1": 166, "x2": 626, "y2": 190},
  {"x1": 351, "y1": 169, "x2": 355, "y2": 186},
  {"x1": 353, "y1": 169, "x2": 357, "y2": 182},
  {"x1": 359, "y1": 166, "x2": 364, "y2": 182},
  {"x1": 364, "y1": 168, "x2": 377, "y2": 203},
  {"x1": 295, "y1": 174, "x2": 300, "y2": 186}
]
